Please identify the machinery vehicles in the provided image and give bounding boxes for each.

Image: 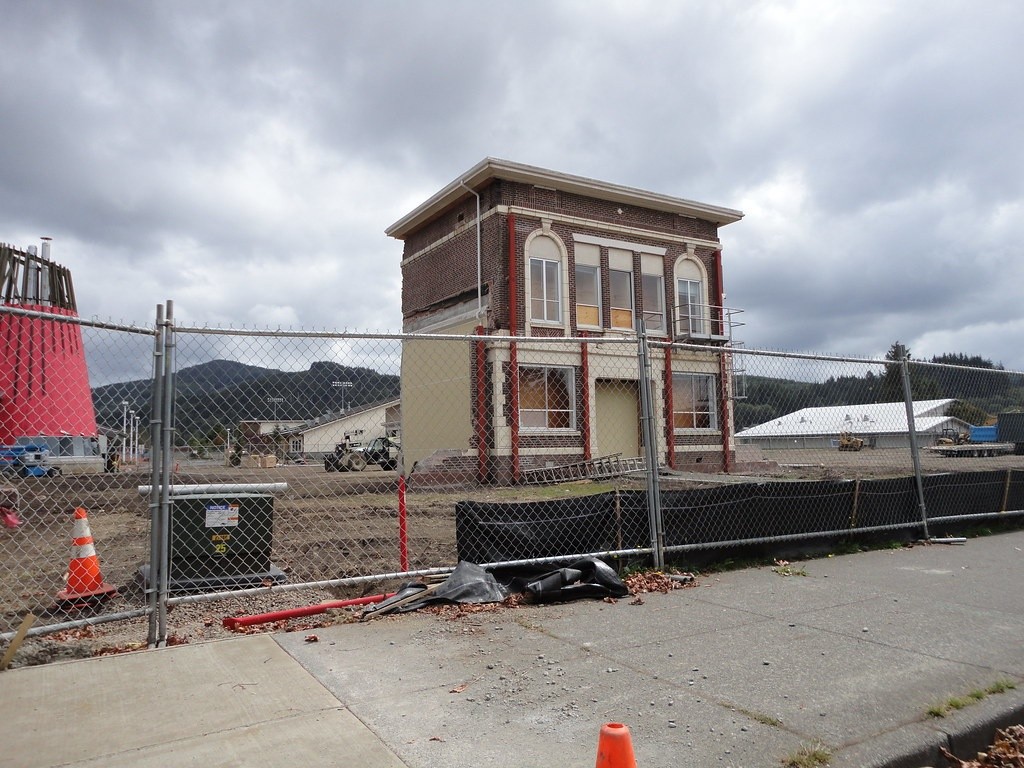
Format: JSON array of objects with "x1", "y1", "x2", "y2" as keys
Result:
[
  {"x1": 839, "y1": 431, "x2": 864, "y2": 451},
  {"x1": 938, "y1": 427, "x2": 971, "y2": 445}
]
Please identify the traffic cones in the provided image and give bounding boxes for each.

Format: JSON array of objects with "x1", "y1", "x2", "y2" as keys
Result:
[
  {"x1": 58, "y1": 507, "x2": 117, "y2": 608},
  {"x1": 596, "y1": 721, "x2": 636, "y2": 768}
]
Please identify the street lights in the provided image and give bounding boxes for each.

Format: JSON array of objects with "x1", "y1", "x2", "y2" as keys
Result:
[
  {"x1": 267, "y1": 397, "x2": 283, "y2": 422},
  {"x1": 134, "y1": 417, "x2": 141, "y2": 461},
  {"x1": 226, "y1": 428, "x2": 230, "y2": 458},
  {"x1": 121, "y1": 400, "x2": 129, "y2": 463},
  {"x1": 332, "y1": 381, "x2": 353, "y2": 410},
  {"x1": 128, "y1": 410, "x2": 135, "y2": 463}
]
